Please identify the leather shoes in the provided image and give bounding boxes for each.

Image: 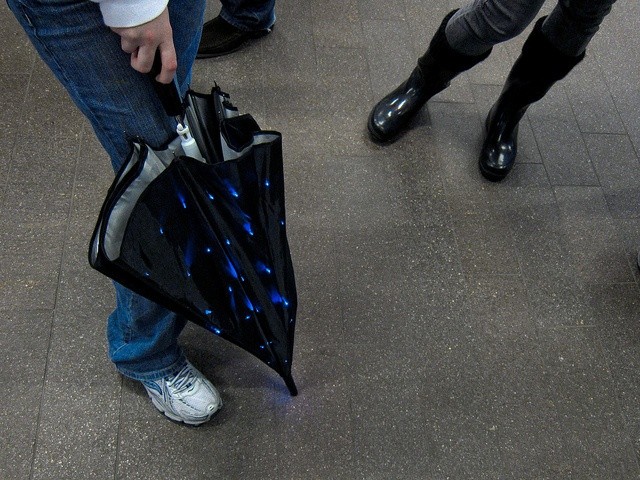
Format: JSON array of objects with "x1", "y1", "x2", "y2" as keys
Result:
[{"x1": 194, "y1": 14, "x2": 274, "y2": 60}]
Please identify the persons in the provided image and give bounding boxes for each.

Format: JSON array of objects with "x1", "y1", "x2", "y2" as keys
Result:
[
  {"x1": 4, "y1": 1, "x2": 224, "y2": 428},
  {"x1": 367, "y1": 0, "x2": 616, "y2": 183},
  {"x1": 193, "y1": 1, "x2": 276, "y2": 60}
]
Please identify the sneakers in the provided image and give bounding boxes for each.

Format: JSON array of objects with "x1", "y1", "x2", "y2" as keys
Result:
[{"x1": 142, "y1": 359, "x2": 222, "y2": 426}]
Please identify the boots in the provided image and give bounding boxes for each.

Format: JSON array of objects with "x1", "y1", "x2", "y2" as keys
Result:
[
  {"x1": 478, "y1": 14, "x2": 585, "y2": 181},
  {"x1": 367, "y1": 9, "x2": 492, "y2": 144}
]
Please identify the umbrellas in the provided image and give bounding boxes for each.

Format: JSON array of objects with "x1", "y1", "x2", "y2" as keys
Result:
[{"x1": 86, "y1": 41, "x2": 299, "y2": 398}]
